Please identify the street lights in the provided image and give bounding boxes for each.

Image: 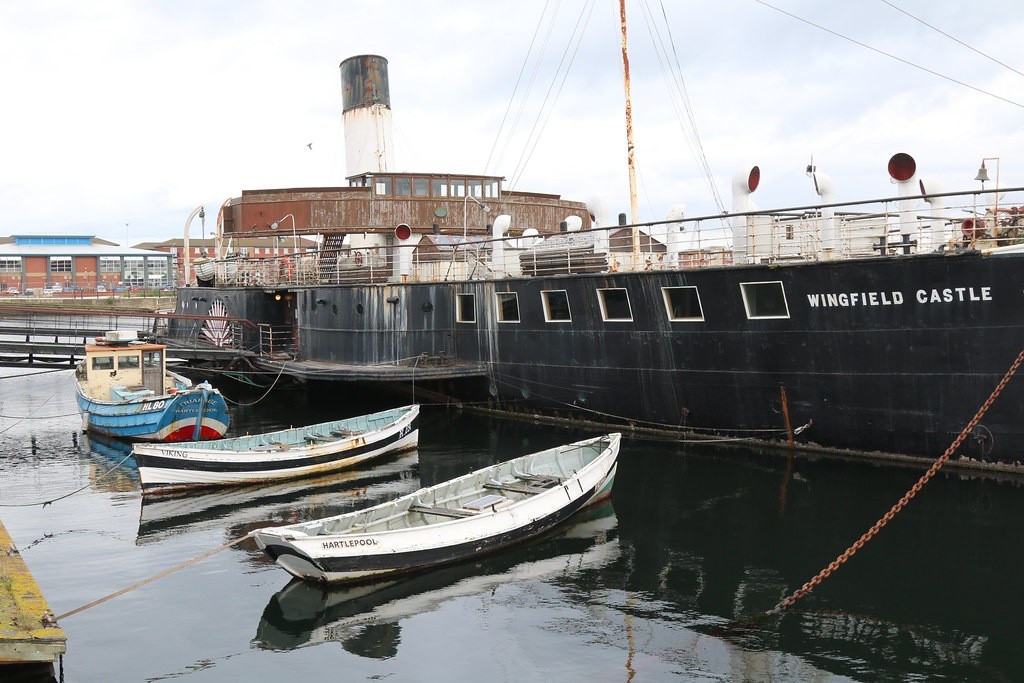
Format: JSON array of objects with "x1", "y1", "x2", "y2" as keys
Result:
[
  {"x1": 271, "y1": 214, "x2": 298, "y2": 285},
  {"x1": 463, "y1": 195, "x2": 492, "y2": 280},
  {"x1": 973, "y1": 157, "x2": 998, "y2": 249}
]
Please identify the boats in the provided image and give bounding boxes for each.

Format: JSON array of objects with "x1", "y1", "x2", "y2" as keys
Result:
[
  {"x1": 134, "y1": 0, "x2": 1024, "y2": 489},
  {"x1": 72, "y1": 331, "x2": 230, "y2": 445},
  {"x1": 248, "y1": 498, "x2": 623, "y2": 653},
  {"x1": 134, "y1": 448, "x2": 421, "y2": 546},
  {"x1": 192, "y1": 255, "x2": 219, "y2": 282},
  {"x1": 135, "y1": 404, "x2": 421, "y2": 495},
  {"x1": 254, "y1": 432, "x2": 623, "y2": 586}
]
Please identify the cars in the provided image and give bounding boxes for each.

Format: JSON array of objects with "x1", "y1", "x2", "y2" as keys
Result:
[{"x1": 1, "y1": 284, "x2": 141, "y2": 296}]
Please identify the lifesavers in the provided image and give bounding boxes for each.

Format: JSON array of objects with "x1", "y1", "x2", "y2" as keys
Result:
[
  {"x1": 95, "y1": 337, "x2": 112, "y2": 346},
  {"x1": 279, "y1": 259, "x2": 293, "y2": 281}
]
